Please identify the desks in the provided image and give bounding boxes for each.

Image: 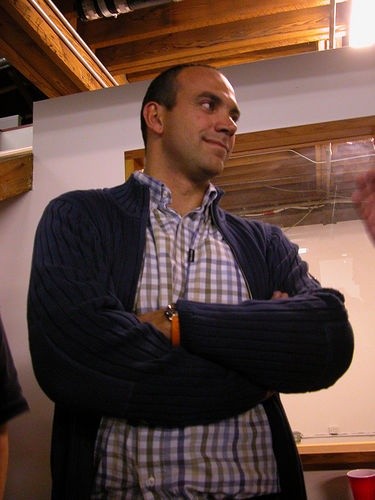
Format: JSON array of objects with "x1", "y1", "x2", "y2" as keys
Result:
[{"x1": 298, "y1": 433, "x2": 375, "y2": 470}]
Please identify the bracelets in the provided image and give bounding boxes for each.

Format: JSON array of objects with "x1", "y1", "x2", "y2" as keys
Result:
[{"x1": 165, "y1": 303, "x2": 181, "y2": 346}]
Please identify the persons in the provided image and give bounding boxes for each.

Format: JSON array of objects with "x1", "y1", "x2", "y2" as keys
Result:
[
  {"x1": 27, "y1": 63, "x2": 355, "y2": 500},
  {"x1": 352, "y1": 168, "x2": 375, "y2": 242},
  {"x1": 0, "y1": 317, "x2": 30, "y2": 500}
]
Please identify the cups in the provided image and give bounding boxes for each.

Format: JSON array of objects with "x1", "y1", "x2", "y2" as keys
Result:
[{"x1": 347, "y1": 469, "x2": 375, "y2": 500}]
